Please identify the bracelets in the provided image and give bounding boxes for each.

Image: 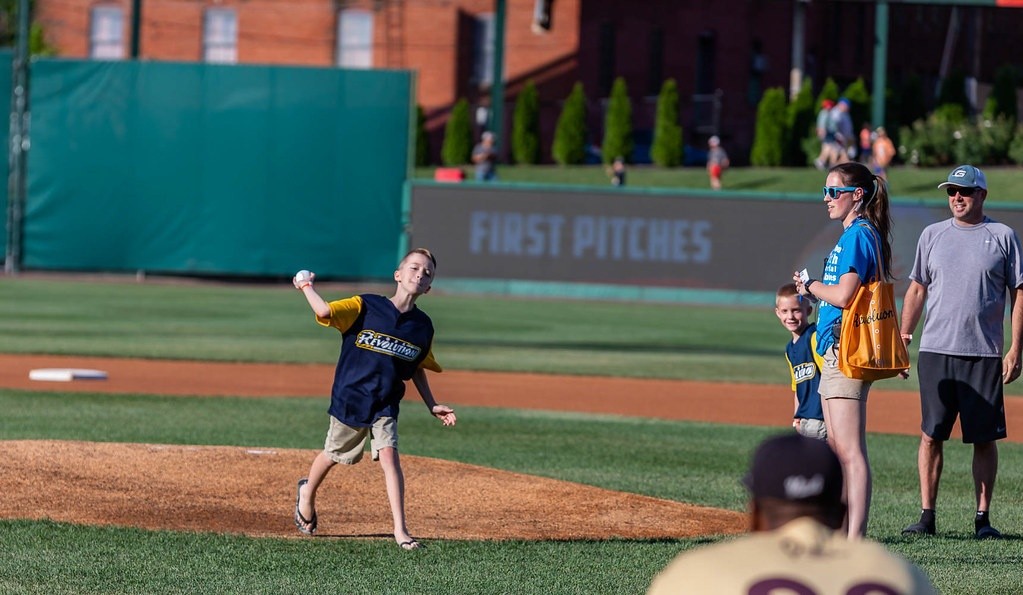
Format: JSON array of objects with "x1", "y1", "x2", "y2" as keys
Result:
[
  {"x1": 901, "y1": 334, "x2": 913, "y2": 345},
  {"x1": 301, "y1": 283, "x2": 313, "y2": 288}
]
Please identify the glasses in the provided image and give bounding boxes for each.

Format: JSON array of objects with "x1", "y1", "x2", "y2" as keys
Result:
[
  {"x1": 947, "y1": 186, "x2": 982, "y2": 197},
  {"x1": 822, "y1": 187, "x2": 868, "y2": 199}
]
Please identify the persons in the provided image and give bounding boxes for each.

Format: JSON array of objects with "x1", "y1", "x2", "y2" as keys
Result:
[
  {"x1": 775, "y1": 284, "x2": 827, "y2": 442},
  {"x1": 813, "y1": 97, "x2": 895, "y2": 181},
  {"x1": 896, "y1": 165, "x2": 1023, "y2": 539},
  {"x1": 648, "y1": 432, "x2": 935, "y2": 595},
  {"x1": 291, "y1": 247, "x2": 456, "y2": 550},
  {"x1": 707, "y1": 136, "x2": 728, "y2": 189},
  {"x1": 792, "y1": 163, "x2": 896, "y2": 536},
  {"x1": 471, "y1": 98, "x2": 499, "y2": 181},
  {"x1": 613, "y1": 159, "x2": 627, "y2": 187}
]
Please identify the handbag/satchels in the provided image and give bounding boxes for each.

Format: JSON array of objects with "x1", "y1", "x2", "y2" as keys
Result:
[{"x1": 839, "y1": 222, "x2": 910, "y2": 382}]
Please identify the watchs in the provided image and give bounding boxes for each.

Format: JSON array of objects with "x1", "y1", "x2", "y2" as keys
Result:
[{"x1": 805, "y1": 279, "x2": 817, "y2": 294}]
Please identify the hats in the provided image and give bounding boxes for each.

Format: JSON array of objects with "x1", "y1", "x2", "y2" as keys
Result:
[
  {"x1": 839, "y1": 97, "x2": 851, "y2": 107},
  {"x1": 823, "y1": 100, "x2": 836, "y2": 108},
  {"x1": 741, "y1": 434, "x2": 843, "y2": 506},
  {"x1": 938, "y1": 165, "x2": 987, "y2": 190}
]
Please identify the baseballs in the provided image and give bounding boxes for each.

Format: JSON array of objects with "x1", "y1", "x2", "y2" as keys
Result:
[{"x1": 296, "y1": 270, "x2": 310, "y2": 283}]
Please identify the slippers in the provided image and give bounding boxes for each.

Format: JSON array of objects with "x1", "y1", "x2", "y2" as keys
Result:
[
  {"x1": 901, "y1": 523, "x2": 936, "y2": 537},
  {"x1": 395, "y1": 537, "x2": 424, "y2": 551},
  {"x1": 295, "y1": 477, "x2": 318, "y2": 536},
  {"x1": 976, "y1": 527, "x2": 1001, "y2": 541}
]
